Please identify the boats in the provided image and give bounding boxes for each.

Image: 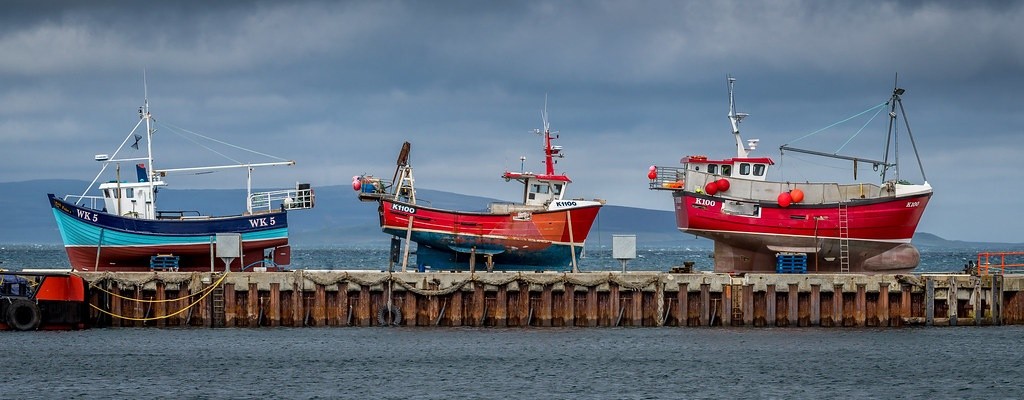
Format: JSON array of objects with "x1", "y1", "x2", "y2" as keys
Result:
[
  {"x1": 649, "y1": 69, "x2": 935, "y2": 273},
  {"x1": 46, "y1": 68, "x2": 317, "y2": 273},
  {"x1": 357, "y1": 93, "x2": 606, "y2": 272}
]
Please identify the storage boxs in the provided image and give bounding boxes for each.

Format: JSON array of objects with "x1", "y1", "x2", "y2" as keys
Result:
[
  {"x1": 149, "y1": 256, "x2": 179, "y2": 272},
  {"x1": 776, "y1": 252, "x2": 807, "y2": 273}
]
